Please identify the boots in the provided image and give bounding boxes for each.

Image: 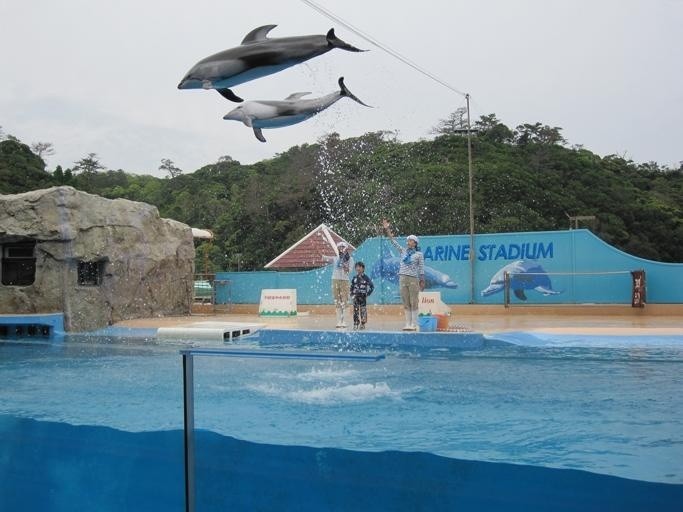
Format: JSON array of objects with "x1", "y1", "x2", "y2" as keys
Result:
[
  {"x1": 403, "y1": 311, "x2": 412, "y2": 330},
  {"x1": 411, "y1": 310, "x2": 419, "y2": 330},
  {"x1": 343, "y1": 307, "x2": 350, "y2": 327},
  {"x1": 335, "y1": 308, "x2": 342, "y2": 328}
]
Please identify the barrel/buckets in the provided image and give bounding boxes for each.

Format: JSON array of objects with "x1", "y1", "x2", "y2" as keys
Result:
[{"x1": 417, "y1": 291, "x2": 450, "y2": 333}]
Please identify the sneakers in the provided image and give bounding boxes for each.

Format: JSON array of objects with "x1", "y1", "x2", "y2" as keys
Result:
[
  {"x1": 360, "y1": 325, "x2": 364, "y2": 329},
  {"x1": 353, "y1": 324, "x2": 358, "y2": 330}
]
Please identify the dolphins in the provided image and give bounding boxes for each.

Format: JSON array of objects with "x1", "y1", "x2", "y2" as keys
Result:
[
  {"x1": 370, "y1": 241, "x2": 459, "y2": 292},
  {"x1": 481, "y1": 258, "x2": 565, "y2": 302},
  {"x1": 176, "y1": 24, "x2": 372, "y2": 103},
  {"x1": 222, "y1": 76, "x2": 375, "y2": 142}
]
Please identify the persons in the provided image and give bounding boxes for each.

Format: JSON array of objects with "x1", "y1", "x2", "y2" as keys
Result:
[
  {"x1": 381, "y1": 218, "x2": 426, "y2": 330},
  {"x1": 313, "y1": 244, "x2": 354, "y2": 329},
  {"x1": 349, "y1": 261, "x2": 374, "y2": 330}
]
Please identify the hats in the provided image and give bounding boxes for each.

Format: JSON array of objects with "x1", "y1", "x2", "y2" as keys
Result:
[
  {"x1": 407, "y1": 235, "x2": 419, "y2": 243},
  {"x1": 337, "y1": 242, "x2": 349, "y2": 249}
]
